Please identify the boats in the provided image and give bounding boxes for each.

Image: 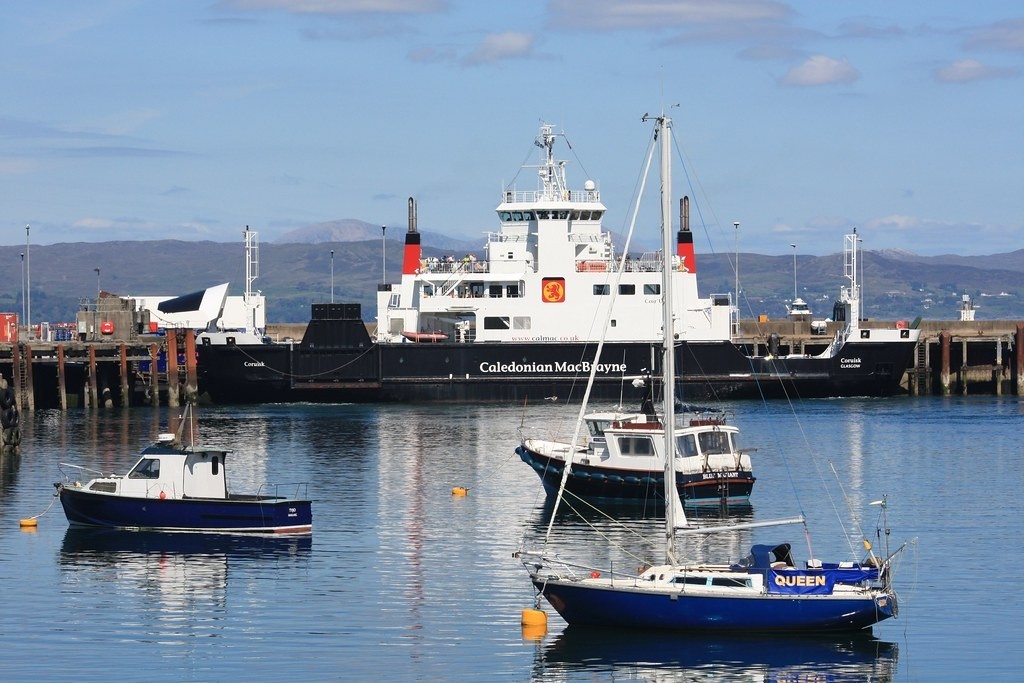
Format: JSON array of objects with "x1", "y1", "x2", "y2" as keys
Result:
[
  {"x1": 744, "y1": 226, "x2": 923, "y2": 395},
  {"x1": 514, "y1": 346, "x2": 758, "y2": 516},
  {"x1": 117, "y1": 225, "x2": 267, "y2": 345},
  {"x1": 375, "y1": 120, "x2": 741, "y2": 343},
  {"x1": 0, "y1": 342, "x2": 190, "y2": 407},
  {"x1": 53, "y1": 393, "x2": 313, "y2": 549},
  {"x1": 0, "y1": 290, "x2": 207, "y2": 358}
]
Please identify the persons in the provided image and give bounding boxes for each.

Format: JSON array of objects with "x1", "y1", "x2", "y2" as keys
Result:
[
  {"x1": 426, "y1": 254, "x2": 476, "y2": 272},
  {"x1": 463, "y1": 286, "x2": 471, "y2": 298}
]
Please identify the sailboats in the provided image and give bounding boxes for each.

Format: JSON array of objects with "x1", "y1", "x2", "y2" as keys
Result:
[{"x1": 512, "y1": 106, "x2": 907, "y2": 641}]
